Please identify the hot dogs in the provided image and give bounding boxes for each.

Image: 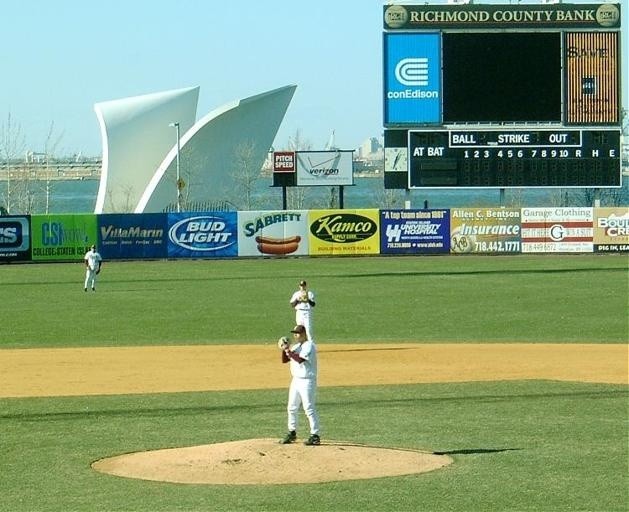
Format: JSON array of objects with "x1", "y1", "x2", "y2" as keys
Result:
[{"x1": 254, "y1": 235, "x2": 300, "y2": 254}]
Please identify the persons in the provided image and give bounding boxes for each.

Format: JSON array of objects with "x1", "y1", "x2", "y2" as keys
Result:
[
  {"x1": 280, "y1": 324, "x2": 320, "y2": 446},
  {"x1": 289, "y1": 279, "x2": 316, "y2": 343},
  {"x1": 83, "y1": 244, "x2": 103, "y2": 292}
]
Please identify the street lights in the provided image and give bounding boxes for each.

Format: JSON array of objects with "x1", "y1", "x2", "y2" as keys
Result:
[{"x1": 168, "y1": 121, "x2": 185, "y2": 215}]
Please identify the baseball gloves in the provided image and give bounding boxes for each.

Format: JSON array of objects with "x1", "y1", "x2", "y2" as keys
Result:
[{"x1": 278, "y1": 336, "x2": 291, "y2": 349}]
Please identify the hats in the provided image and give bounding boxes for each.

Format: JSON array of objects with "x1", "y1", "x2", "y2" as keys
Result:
[
  {"x1": 290, "y1": 325, "x2": 306, "y2": 333},
  {"x1": 91, "y1": 245, "x2": 96, "y2": 248},
  {"x1": 299, "y1": 280, "x2": 307, "y2": 286}
]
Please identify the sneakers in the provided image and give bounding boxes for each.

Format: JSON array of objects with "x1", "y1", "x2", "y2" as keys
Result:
[
  {"x1": 279, "y1": 430, "x2": 297, "y2": 444},
  {"x1": 84, "y1": 287, "x2": 95, "y2": 292},
  {"x1": 303, "y1": 433, "x2": 321, "y2": 446}
]
